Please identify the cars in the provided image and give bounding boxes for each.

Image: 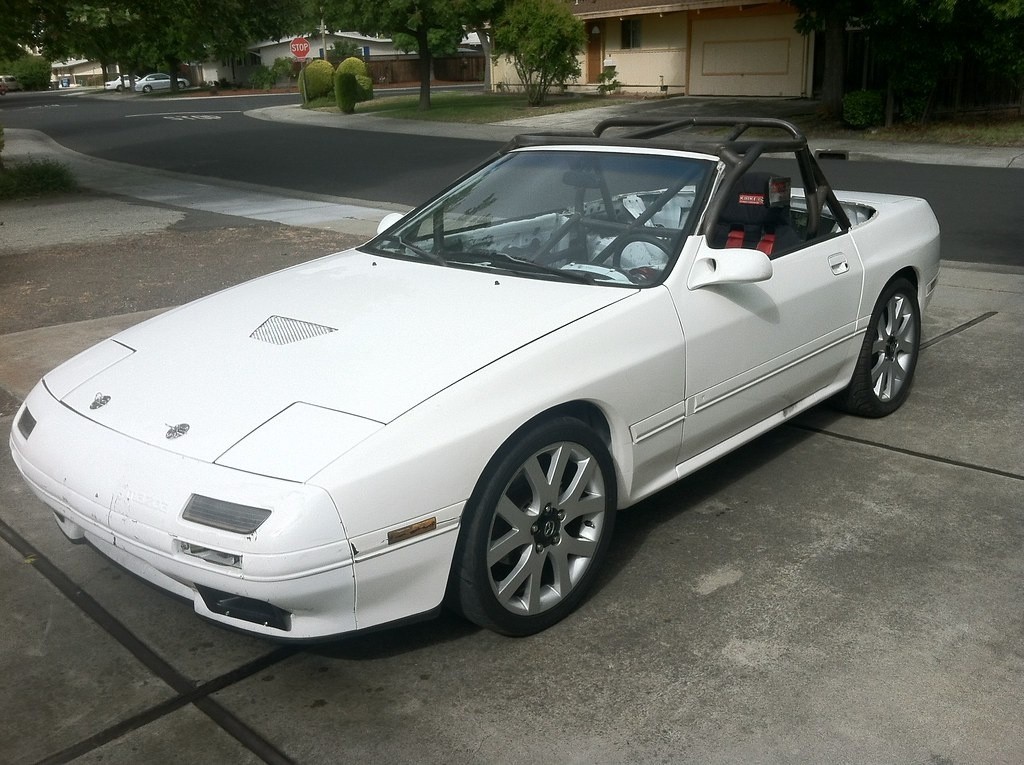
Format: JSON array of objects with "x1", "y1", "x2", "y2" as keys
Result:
[
  {"x1": 105, "y1": 74, "x2": 142, "y2": 92},
  {"x1": 135, "y1": 73, "x2": 189, "y2": 94},
  {"x1": 61, "y1": 78, "x2": 70, "y2": 87},
  {"x1": 0, "y1": 75, "x2": 23, "y2": 91},
  {"x1": 0, "y1": 82, "x2": 8, "y2": 95}
]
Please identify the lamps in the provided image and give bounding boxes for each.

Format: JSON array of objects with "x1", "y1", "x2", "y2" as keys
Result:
[{"x1": 592, "y1": 25, "x2": 600, "y2": 33}]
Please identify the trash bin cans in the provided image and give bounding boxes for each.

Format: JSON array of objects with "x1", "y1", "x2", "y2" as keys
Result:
[
  {"x1": 50, "y1": 81, "x2": 59, "y2": 90},
  {"x1": 62, "y1": 78, "x2": 69, "y2": 87}
]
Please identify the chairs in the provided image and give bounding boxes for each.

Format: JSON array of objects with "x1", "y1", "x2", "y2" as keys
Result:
[{"x1": 719, "y1": 170, "x2": 796, "y2": 256}]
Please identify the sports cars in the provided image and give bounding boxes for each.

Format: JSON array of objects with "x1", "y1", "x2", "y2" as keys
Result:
[{"x1": 9, "y1": 117, "x2": 943, "y2": 644}]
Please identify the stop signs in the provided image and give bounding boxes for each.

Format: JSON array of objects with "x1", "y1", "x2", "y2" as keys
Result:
[{"x1": 290, "y1": 38, "x2": 310, "y2": 62}]
